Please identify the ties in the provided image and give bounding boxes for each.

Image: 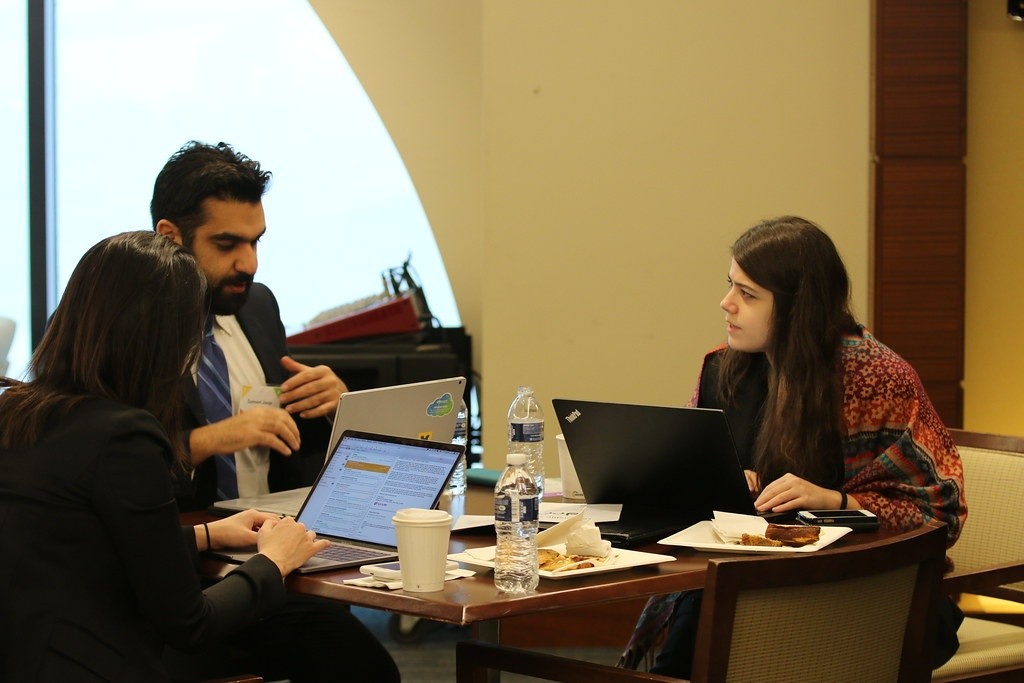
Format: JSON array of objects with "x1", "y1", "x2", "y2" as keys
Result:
[{"x1": 197, "y1": 336, "x2": 239, "y2": 511}]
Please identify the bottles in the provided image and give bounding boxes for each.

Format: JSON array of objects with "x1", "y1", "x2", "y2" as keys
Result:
[
  {"x1": 494, "y1": 453, "x2": 540, "y2": 593},
  {"x1": 506, "y1": 385, "x2": 545, "y2": 498},
  {"x1": 443, "y1": 395, "x2": 467, "y2": 495}
]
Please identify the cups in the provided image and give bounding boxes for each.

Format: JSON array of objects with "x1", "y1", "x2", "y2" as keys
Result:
[
  {"x1": 391, "y1": 507, "x2": 454, "y2": 593},
  {"x1": 555, "y1": 434, "x2": 586, "y2": 499}
]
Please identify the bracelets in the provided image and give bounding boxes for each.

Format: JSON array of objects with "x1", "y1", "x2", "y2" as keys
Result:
[
  {"x1": 840, "y1": 490, "x2": 848, "y2": 510},
  {"x1": 203, "y1": 522, "x2": 211, "y2": 549}
]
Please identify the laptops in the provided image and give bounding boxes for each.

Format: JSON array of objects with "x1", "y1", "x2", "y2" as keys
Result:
[
  {"x1": 201, "y1": 429, "x2": 465, "y2": 573},
  {"x1": 213, "y1": 376, "x2": 466, "y2": 521},
  {"x1": 552, "y1": 399, "x2": 800, "y2": 526}
]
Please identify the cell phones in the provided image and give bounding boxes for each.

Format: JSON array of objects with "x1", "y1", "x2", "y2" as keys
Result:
[{"x1": 795, "y1": 510, "x2": 881, "y2": 532}]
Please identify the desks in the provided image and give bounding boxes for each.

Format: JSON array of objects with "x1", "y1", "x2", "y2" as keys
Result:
[{"x1": 173, "y1": 473, "x2": 903, "y2": 683}]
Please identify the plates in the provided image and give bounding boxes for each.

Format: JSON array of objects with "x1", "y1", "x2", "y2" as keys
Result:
[
  {"x1": 447, "y1": 542, "x2": 677, "y2": 581},
  {"x1": 657, "y1": 520, "x2": 853, "y2": 553}
]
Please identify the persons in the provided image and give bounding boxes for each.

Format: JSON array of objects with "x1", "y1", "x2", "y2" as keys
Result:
[
  {"x1": 152, "y1": 145, "x2": 401, "y2": 682},
  {"x1": 614, "y1": 217, "x2": 966, "y2": 680},
  {"x1": 0, "y1": 231, "x2": 332, "y2": 683}
]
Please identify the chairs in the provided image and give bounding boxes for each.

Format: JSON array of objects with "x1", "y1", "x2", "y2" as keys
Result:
[
  {"x1": 451, "y1": 518, "x2": 950, "y2": 681},
  {"x1": 928, "y1": 429, "x2": 1024, "y2": 683}
]
